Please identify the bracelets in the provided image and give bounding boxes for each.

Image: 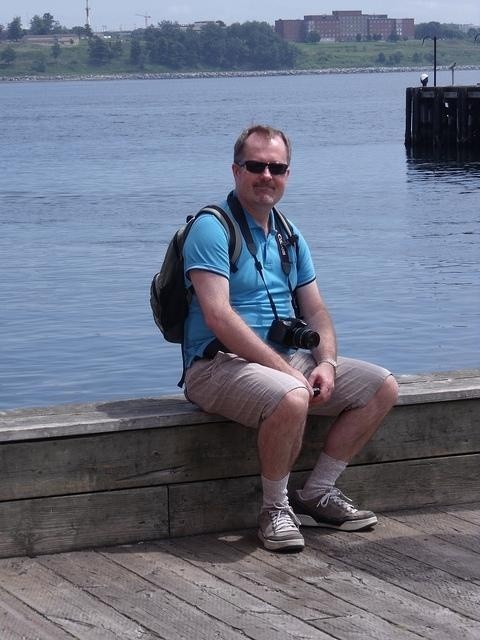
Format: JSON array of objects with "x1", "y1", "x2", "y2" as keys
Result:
[{"x1": 318, "y1": 358, "x2": 339, "y2": 376}]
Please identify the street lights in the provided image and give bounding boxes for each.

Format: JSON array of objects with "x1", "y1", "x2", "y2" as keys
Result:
[{"x1": 422, "y1": 35, "x2": 436, "y2": 87}]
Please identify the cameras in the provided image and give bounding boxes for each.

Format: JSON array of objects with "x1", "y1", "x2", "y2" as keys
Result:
[{"x1": 269, "y1": 320, "x2": 320, "y2": 351}]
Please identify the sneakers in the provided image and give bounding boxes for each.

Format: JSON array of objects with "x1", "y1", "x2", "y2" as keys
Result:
[
  {"x1": 256, "y1": 501, "x2": 306, "y2": 553},
  {"x1": 292, "y1": 486, "x2": 379, "y2": 532}
]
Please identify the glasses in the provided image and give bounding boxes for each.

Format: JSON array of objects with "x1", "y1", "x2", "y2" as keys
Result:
[{"x1": 240, "y1": 160, "x2": 289, "y2": 175}]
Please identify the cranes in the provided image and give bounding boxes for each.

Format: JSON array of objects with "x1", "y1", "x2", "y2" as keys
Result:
[{"x1": 135, "y1": 13, "x2": 152, "y2": 26}]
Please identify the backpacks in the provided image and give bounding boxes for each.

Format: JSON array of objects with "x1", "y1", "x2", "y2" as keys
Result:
[{"x1": 150, "y1": 203, "x2": 298, "y2": 345}]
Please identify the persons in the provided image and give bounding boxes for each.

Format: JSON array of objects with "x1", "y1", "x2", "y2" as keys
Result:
[{"x1": 182, "y1": 125, "x2": 400, "y2": 551}]
